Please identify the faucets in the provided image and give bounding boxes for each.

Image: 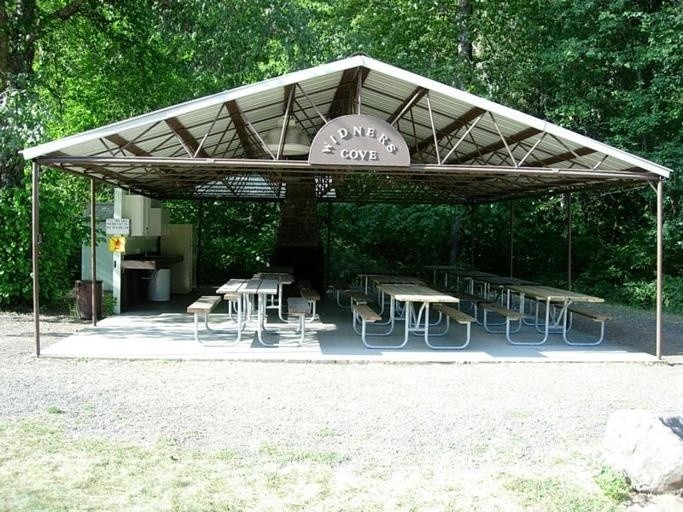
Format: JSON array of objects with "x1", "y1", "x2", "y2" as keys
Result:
[{"x1": 145, "y1": 247, "x2": 151, "y2": 257}]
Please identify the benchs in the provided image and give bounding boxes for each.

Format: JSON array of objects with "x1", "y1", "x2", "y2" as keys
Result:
[
  {"x1": 435, "y1": 268, "x2": 614, "y2": 349},
  {"x1": 186, "y1": 272, "x2": 321, "y2": 349},
  {"x1": 329, "y1": 271, "x2": 478, "y2": 348}
]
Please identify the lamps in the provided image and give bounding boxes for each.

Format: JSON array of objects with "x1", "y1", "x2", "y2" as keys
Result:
[{"x1": 261, "y1": 112, "x2": 311, "y2": 157}]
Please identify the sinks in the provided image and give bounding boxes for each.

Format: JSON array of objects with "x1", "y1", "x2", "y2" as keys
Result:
[{"x1": 148, "y1": 256, "x2": 175, "y2": 258}]
